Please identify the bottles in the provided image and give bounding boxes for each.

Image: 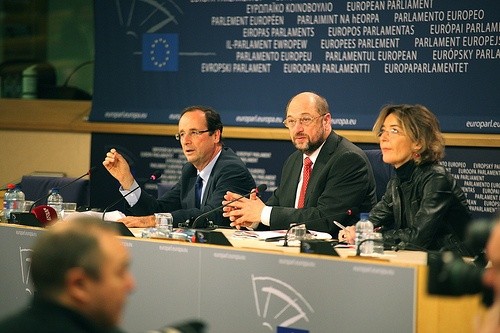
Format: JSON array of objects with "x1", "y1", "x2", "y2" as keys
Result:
[
  {"x1": 354, "y1": 212, "x2": 375, "y2": 255},
  {"x1": 3, "y1": 184, "x2": 15, "y2": 219},
  {"x1": 13, "y1": 184, "x2": 26, "y2": 215},
  {"x1": 47, "y1": 187, "x2": 63, "y2": 219}
]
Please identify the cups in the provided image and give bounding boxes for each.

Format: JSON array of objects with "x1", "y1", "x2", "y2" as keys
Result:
[
  {"x1": 289, "y1": 223, "x2": 307, "y2": 243},
  {"x1": 62, "y1": 202, "x2": 77, "y2": 215},
  {"x1": 364, "y1": 233, "x2": 384, "y2": 255},
  {"x1": 155, "y1": 216, "x2": 174, "y2": 231}
]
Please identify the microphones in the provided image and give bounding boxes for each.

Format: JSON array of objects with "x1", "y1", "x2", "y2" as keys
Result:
[
  {"x1": 29, "y1": 167, "x2": 98, "y2": 213},
  {"x1": 191, "y1": 184, "x2": 267, "y2": 229},
  {"x1": 0, "y1": 183, "x2": 23, "y2": 191},
  {"x1": 102, "y1": 171, "x2": 161, "y2": 222},
  {"x1": 284, "y1": 208, "x2": 360, "y2": 247}
]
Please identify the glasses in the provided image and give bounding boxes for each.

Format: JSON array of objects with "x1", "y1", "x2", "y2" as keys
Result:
[
  {"x1": 282, "y1": 113, "x2": 326, "y2": 128},
  {"x1": 376, "y1": 128, "x2": 408, "y2": 138},
  {"x1": 175, "y1": 128, "x2": 211, "y2": 141}
]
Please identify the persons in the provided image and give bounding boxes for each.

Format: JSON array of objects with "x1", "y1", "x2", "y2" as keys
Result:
[
  {"x1": 338, "y1": 104, "x2": 474, "y2": 253},
  {"x1": 222, "y1": 92, "x2": 377, "y2": 245},
  {"x1": 0, "y1": 213, "x2": 184, "y2": 333},
  {"x1": 102, "y1": 106, "x2": 256, "y2": 228}
]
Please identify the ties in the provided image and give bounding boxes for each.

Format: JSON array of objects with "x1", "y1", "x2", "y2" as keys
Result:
[
  {"x1": 194, "y1": 176, "x2": 203, "y2": 209},
  {"x1": 297, "y1": 157, "x2": 313, "y2": 209}
]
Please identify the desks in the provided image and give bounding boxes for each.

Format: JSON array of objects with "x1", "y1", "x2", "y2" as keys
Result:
[{"x1": 0, "y1": 222, "x2": 494, "y2": 333}]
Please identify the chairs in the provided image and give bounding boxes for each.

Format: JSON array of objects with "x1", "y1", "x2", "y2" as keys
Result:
[
  {"x1": 363, "y1": 149, "x2": 393, "y2": 203},
  {"x1": 18, "y1": 176, "x2": 90, "y2": 209}
]
